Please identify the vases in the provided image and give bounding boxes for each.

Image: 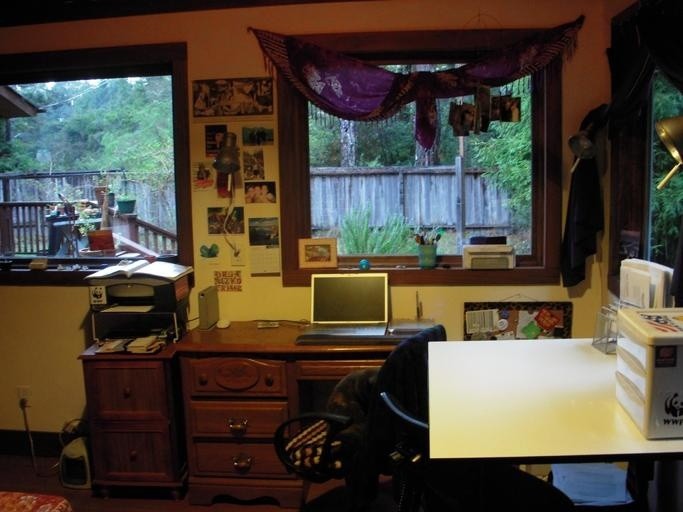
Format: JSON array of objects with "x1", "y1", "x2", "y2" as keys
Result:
[
  {"x1": 117, "y1": 200, "x2": 136, "y2": 213},
  {"x1": 79, "y1": 248, "x2": 104, "y2": 257}
]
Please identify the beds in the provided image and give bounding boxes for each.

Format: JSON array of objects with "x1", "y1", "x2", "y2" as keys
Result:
[{"x1": 0, "y1": 492, "x2": 72, "y2": 512}]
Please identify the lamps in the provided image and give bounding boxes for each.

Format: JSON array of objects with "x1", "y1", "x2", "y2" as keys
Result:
[
  {"x1": 212, "y1": 132, "x2": 240, "y2": 256},
  {"x1": 654, "y1": 117, "x2": 683, "y2": 308}
]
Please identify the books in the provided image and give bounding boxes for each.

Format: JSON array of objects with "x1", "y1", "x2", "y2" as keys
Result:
[
  {"x1": 96, "y1": 335, "x2": 163, "y2": 353},
  {"x1": 83, "y1": 259, "x2": 193, "y2": 283},
  {"x1": 619, "y1": 257, "x2": 676, "y2": 310}
]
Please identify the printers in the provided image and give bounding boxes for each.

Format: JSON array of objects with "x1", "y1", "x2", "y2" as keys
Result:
[{"x1": 88, "y1": 266, "x2": 193, "y2": 353}]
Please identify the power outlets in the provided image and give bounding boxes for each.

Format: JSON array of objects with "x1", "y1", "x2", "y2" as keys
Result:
[{"x1": 16, "y1": 385, "x2": 32, "y2": 407}]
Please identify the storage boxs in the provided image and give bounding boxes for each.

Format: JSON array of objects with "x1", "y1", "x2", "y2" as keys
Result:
[{"x1": 614, "y1": 308, "x2": 683, "y2": 439}]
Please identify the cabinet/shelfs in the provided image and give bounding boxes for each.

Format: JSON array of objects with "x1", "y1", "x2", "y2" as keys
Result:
[
  {"x1": 175, "y1": 322, "x2": 435, "y2": 509},
  {"x1": 77, "y1": 342, "x2": 188, "y2": 502}
]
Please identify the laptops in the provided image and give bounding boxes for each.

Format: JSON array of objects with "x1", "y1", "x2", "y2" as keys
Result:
[{"x1": 301, "y1": 272, "x2": 388, "y2": 336}]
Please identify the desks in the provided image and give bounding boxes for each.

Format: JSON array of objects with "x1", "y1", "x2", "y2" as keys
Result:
[{"x1": 428, "y1": 338, "x2": 683, "y2": 464}]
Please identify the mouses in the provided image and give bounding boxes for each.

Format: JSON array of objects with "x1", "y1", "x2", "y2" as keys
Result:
[{"x1": 217, "y1": 319, "x2": 230, "y2": 328}]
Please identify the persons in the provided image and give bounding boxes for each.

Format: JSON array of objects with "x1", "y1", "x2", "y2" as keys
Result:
[{"x1": 245, "y1": 184, "x2": 276, "y2": 203}]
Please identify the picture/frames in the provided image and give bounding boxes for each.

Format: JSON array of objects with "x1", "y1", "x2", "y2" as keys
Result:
[
  {"x1": 298, "y1": 238, "x2": 337, "y2": 269},
  {"x1": 463, "y1": 302, "x2": 572, "y2": 340}
]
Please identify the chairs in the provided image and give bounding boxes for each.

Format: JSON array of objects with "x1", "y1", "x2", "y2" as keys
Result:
[
  {"x1": 379, "y1": 324, "x2": 446, "y2": 509},
  {"x1": 274, "y1": 367, "x2": 382, "y2": 511}
]
